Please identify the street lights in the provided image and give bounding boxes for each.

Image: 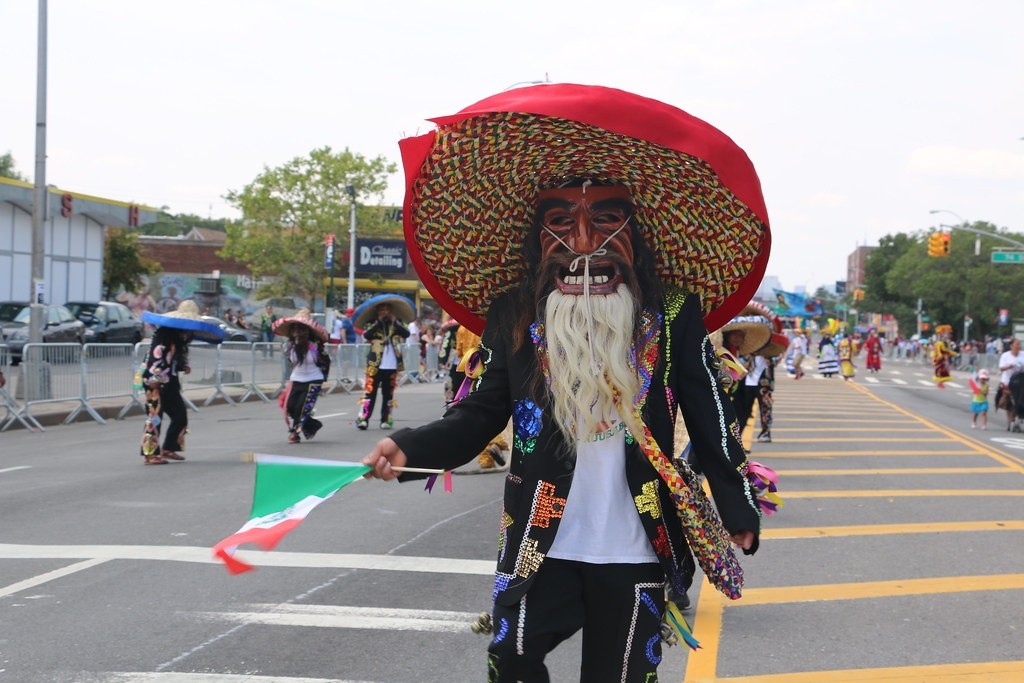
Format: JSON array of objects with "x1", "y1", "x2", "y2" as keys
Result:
[
  {"x1": 345, "y1": 182, "x2": 357, "y2": 316},
  {"x1": 929, "y1": 210, "x2": 973, "y2": 342}
]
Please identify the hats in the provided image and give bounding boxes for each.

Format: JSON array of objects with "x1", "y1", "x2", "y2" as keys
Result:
[
  {"x1": 271, "y1": 307, "x2": 328, "y2": 344},
  {"x1": 140, "y1": 298, "x2": 226, "y2": 345},
  {"x1": 760, "y1": 333, "x2": 786, "y2": 360},
  {"x1": 401, "y1": 85, "x2": 771, "y2": 339},
  {"x1": 977, "y1": 369, "x2": 991, "y2": 380},
  {"x1": 350, "y1": 296, "x2": 419, "y2": 331},
  {"x1": 709, "y1": 316, "x2": 772, "y2": 355}
]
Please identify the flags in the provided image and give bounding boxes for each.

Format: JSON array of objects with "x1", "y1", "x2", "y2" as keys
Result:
[{"x1": 212, "y1": 458, "x2": 371, "y2": 574}]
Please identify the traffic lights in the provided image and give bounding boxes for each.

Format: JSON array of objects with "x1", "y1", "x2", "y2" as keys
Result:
[
  {"x1": 928, "y1": 235, "x2": 950, "y2": 257},
  {"x1": 922, "y1": 323, "x2": 928, "y2": 332},
  {"x1": 852, "y1": 289, "x2": 865, "y2": 301}
]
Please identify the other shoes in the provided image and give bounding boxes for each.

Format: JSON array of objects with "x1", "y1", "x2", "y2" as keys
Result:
[
  {"x1": 359, "y1": 420, "x2": 367, "y2": 429},
  {"x1": 161, "y1": 450, "x2": 184, "y2": 460},
  {"x1": 287, "y1": 432, "x2": 301, "y2": 442},
  {"x1": 487, "y1": 446, "x2": 506, "y2": 465},
  {"x1": 143, "y1": 454, "x2": 165, "y2": 464},
  {"x1": 381, "y1": 421, "x2": 391, "y2": 429}
]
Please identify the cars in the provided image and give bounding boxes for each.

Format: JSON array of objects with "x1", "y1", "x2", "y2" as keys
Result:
[
  {"x1": 0, "y1": 303, "x2": 87, "y2": 365},
  {"x1": 61, "y1": 301, "x2": 144, "y2": 356}
]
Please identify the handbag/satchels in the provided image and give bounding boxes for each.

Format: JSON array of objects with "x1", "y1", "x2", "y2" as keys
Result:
[{"x1": 668, "y1": 456, "x2": 745, "y2": 601}]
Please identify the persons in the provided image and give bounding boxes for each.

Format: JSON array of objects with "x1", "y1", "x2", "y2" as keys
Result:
[
  {"x1": 933, "y1": 333, "x2": 957, "y2": 388},
  {"x1": 971, "y1": 369, "x2": 989, "y2": 430},
  {"x1": 995, "y1": 338, "x2": 1024, "y2": 430},
  {"x1": 329, "y1": 304, "x2": 444, "y2": 381},
  {"x1": 721, "y1": 301, "x2": 788, "y2": 452},
  {"x1": 270, "y1": 308, "x2": 329, "y2": 443},
  {"x1": 138, "y1": 300, "x2": 224, "y2": 464},
  {"x1": 352, "y1": 294, "x2": 417, "y2": 428},
  {"x1": 785, "y1": 327, "x2": 811, "y2": 379},
  {"x1": 439, "y1": 317, "x2": 510, "y2": 470},
  {"x1": 818, "y1": 327, "x2": 883, "y2": 380},
  {"x1": 226, "y1": 305, "x2": 276, "y2": 359},
  {"x1": 892, "y1": 337, "x2": 994, "y2": 372},
  {"x1": 365, "y1": 85, "x2": 771, "y2": 683}
]
